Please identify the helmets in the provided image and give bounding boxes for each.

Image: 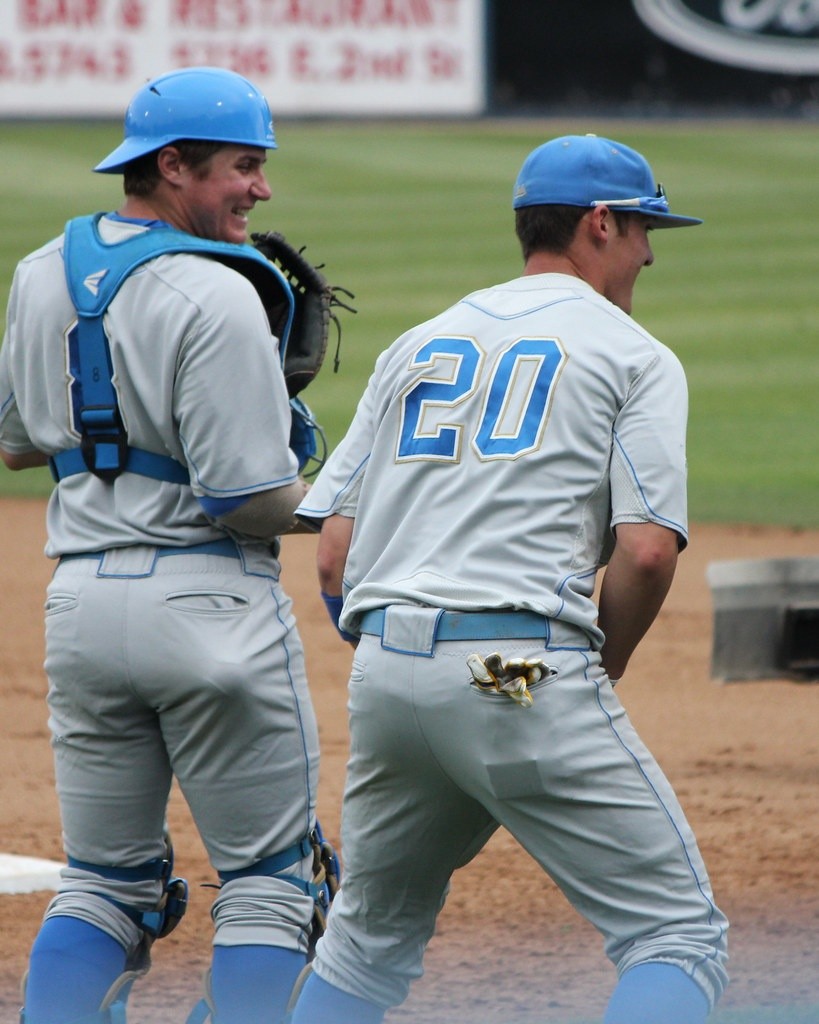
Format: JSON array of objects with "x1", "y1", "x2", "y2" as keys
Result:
[{"x1": 93, "y1": 68, "x2": 276, "y2": 173}]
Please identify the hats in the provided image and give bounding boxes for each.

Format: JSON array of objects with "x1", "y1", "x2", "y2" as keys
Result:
[{"x1": 516, "y1": 133, "x2": 705, "y2": 227}]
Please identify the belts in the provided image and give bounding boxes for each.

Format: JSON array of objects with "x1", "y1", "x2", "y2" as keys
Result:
[
  {"x1": 358, "y1": 606, "x2": 549, "y2": 643},
  {"x1": 59, "y1": 540, "x2": 241, "y2": 561}
]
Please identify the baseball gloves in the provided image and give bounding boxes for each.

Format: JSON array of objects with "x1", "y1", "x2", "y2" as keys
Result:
[{"x1": 247, "y1": 226, "x2": 330, "y2": 399}]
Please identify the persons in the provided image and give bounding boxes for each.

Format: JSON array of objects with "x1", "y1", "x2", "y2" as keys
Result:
[
  {"x1": 1, "y1": 64, "x2": 327, "y2": 1024},
  {"x1": 287, "y1": 132, "x2": 736, "y2": 1024}
]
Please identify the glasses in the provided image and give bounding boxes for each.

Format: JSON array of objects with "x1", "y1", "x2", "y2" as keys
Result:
[{"x1": 591, "y1": 184, "x2": 669, "y2": 212}]
